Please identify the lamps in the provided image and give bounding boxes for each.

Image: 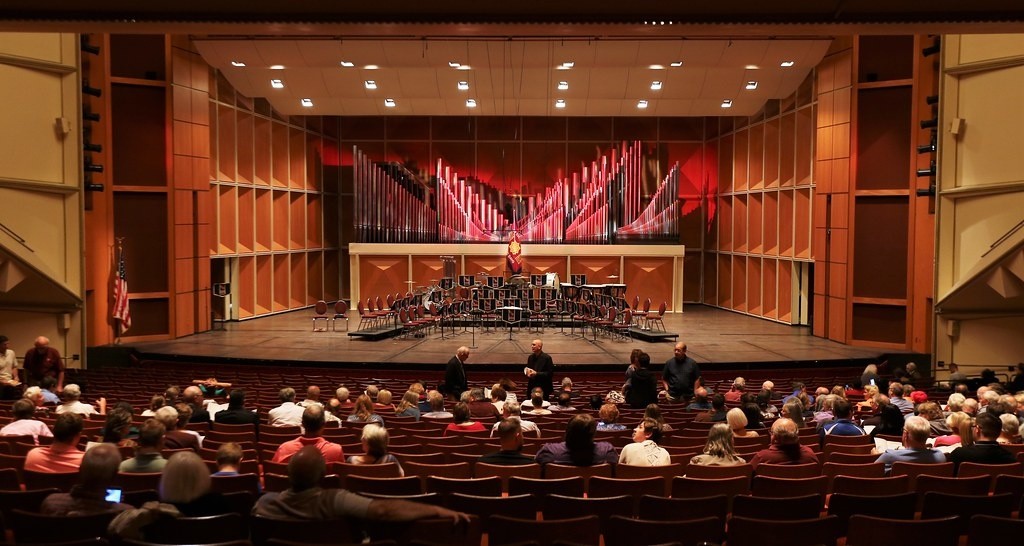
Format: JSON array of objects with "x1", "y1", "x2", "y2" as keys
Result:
[
  {"x1": 81, "y1": 42, "x2": 100, "y2": 55},
  {"x1": 84, "y1": 156, "x2": 103, "y2": 173},
  {"x1": 83, "y1": 111, "x2": 101, "y2": 123},
  {"x1": 84, "y1": 180, "x2": 105, "y2": 191},
  {"x1": 916, "y1": 182, "x2": 936, "y2": 196},
  {"x1": 82, "y1": 84, "x2": 103, "y2": 98},
  {"x1": 916, "y1": 133, "x2": 937, "y2": 155},
  {"x1": 927, "y1": 94, "x2": 938, "y2": 105},
  {"x1": 922, "y1": 35, "x2": 940, "y2": 58},
  {"x1": 83, "y1": 132, "x2": 102, "y2": 153},
  {"x1": 920, "y1": 116, "x2": 938, "y2": 129},
  {"x1": 916, "y1": 159, "x2": 936, "y2": 178}
]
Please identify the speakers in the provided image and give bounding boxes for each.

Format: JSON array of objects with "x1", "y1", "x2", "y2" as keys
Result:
[
  {"x1": 56, "y1": 117, "x2": 69, "y2": 134},
  {"x1": 951, "y1": 117, "x2": 966, "y2": 134}
]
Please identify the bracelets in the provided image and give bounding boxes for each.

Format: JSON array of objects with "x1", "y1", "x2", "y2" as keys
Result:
[{"x1": 23, "y1": 383, "x2": 28, "y2": 384}]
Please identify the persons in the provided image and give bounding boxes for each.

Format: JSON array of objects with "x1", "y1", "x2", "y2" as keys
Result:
[
  {"x1": 0, "y1": 335, "x2": 20, "y2": 400},
  {"x1": 860, "y1": 356, "x2": 888, "y2": 388},
  {"x1": 526, "y1": 339, "x2": 555, "y2": 401},
  {"x1": 624, "y1": 349, "x2": 643, "y2": 381},
  {"x1": 661, "y1": 343, "x2": 705, "y2": 404},
  {"x1": 905, "y1": 362, "x2": 922, "y2": 378},
  {"x1": 1008, "y1": 363, "x2": 1024, "y2": 395},
  {"x1": 981, "y1": 369, "x2": 999, "y2": 385},
  {"x1": 22, "y1": 336, "x2": 65, "y2": 396},
  {"x1": 949, "y1": 363, "x2": 967, "y2": 380},
  {"x1": 630, "y1": 353, "x2": 658, "y2": 408},
  {"x1": 0, "y1": 377, "x2": 1024, "y2": 546},
  {"x1": 892, "y1": 367, "x2": 909, "y2": 385},
  {"x1": 445, "y1": 346, "x2": 469, "y2": 401}
]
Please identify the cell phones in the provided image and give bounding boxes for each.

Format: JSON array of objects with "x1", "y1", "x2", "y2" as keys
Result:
[
  {"x1": 846, "y1": 385, "x2": 849, "y2": 388},
  {"x1": 870, "y1": 379, "x2": 875, "y2": 385}
]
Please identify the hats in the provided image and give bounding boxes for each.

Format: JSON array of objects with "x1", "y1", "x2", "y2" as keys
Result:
[{"x1": 910, "y1": 390, "x2": 928, "y2": 403}]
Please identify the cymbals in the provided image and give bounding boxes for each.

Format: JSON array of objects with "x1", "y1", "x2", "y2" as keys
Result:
[
  {"x1": 404, "y1": 281, "x2": 417, "y2": 284},
  {"x1": 430, "y1": 280, "x2": 438, "y2": 282},
  {"x1": 415, "y1": 286, "x2": 427, "y2": 289},
  {"x1": 606, "y1": 275, "x2": 619, "y2": 278}
]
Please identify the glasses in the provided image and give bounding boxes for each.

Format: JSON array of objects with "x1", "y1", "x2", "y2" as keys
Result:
[
  {"x1": 768, "y1": 430, "x2": 776, "y2": 437},
  {"x1": 915, "y1": 411, "x2": 925, "y2": 416},
  {"x1": 970, "y1": 426, "x2": 982, "y2": 431}
]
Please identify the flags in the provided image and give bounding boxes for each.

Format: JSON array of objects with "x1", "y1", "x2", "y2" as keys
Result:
[{"x1": 111, "y1": 255, "x2": 131, "y2": 335}]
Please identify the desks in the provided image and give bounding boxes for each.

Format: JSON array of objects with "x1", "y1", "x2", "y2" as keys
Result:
[{"x1": 559, "y1": 283, "x2": 627, "y2": 307}]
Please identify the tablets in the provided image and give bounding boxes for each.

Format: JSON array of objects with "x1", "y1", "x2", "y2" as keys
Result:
[{"x1": 103, "y1": 487, "x2": 122, "y2": 502}]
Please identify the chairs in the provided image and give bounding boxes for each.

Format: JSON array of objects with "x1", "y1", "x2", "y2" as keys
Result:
[
  {"x1": 0, "y1": 362, "x2": 1024, "y2": 546},
  {"x1": 311, "y1": 300, "x2": 330, "y2": 331},
  {"x1": 358, "y1": 286, "x2": 667, "y2": 343},
  {"x1": 332, "y1": 300, "x2": 349, "y2": 331}
]
